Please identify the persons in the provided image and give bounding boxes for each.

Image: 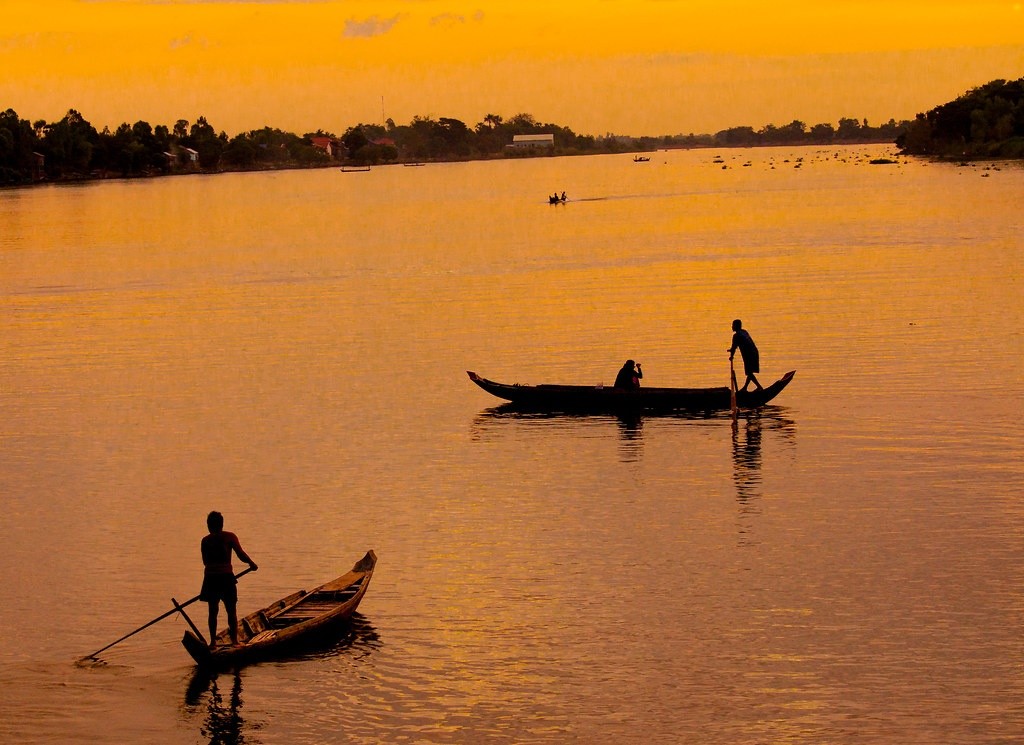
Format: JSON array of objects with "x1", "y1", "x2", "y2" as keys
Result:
[
  {"x1": 614, "y1": 359, "x2": 642, "y2": 387},
  {"x1": 199, "y1": 510, "x2": 259, "y2": 649},
  {"x1": 729, "y1": 319, "x2": 764, "y2": 392}
]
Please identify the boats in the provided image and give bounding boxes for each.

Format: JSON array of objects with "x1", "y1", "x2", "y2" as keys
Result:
[
  {"x1": 467, "y1": 370, "x2": 796, "y2": 413},
  {"x1": 183, "y1": 551, "x2": 377, "y2": 671}
]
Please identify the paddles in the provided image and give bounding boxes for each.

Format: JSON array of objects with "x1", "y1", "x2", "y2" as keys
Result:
[
  {"x1": 561, "y1": 191, "x2": 571, "y2": 201},
  {"x1": 728, "y1": 349, "x2": 740, "y2": 421},
  {"x1": 82, "y1": 564, "x2": 254, "y2": 659}
]
